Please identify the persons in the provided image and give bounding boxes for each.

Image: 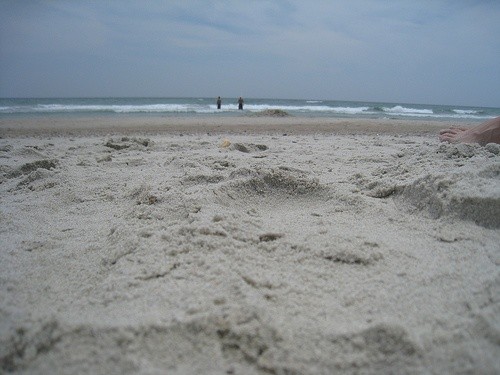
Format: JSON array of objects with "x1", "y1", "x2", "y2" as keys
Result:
[
  {"x1": 217, "y1": 96, "x2": 221, "y2": 110},
  {"x1": 238, "y1": 96, "x2": 244, "y2": 110}
]
